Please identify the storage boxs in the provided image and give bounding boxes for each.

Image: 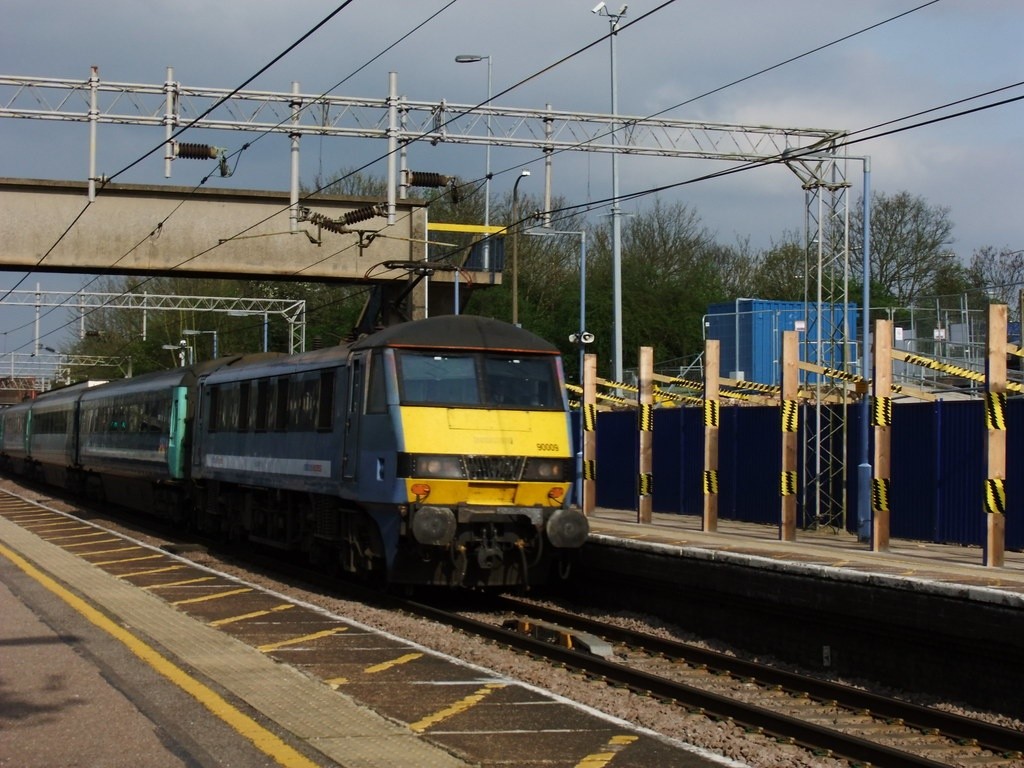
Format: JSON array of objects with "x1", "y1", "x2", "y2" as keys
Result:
[{"x1": 708, "y1": 299, "x2": 860, "y2": 386}]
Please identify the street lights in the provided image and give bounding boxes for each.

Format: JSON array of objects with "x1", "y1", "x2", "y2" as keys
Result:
[
  {"x1": 455, "y1": 55, "x2": 492, "y2": 271},
  {"x1": 162, "y1": 345, "x2": 192, "y2": 365},
  {"x1": 521, "y1": 226, "x2": 585, "y2": 509},
  {"x1": 782, "y1": 147, "x2": 872, "y2": 544},
  {"x1": 182, "y1": 330, "x2": 217, "y2": 360}
]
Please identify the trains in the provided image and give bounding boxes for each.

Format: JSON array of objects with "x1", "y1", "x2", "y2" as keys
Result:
[{"x1": 0, "y1": 315, "x2": 590, "y2": 608}]
